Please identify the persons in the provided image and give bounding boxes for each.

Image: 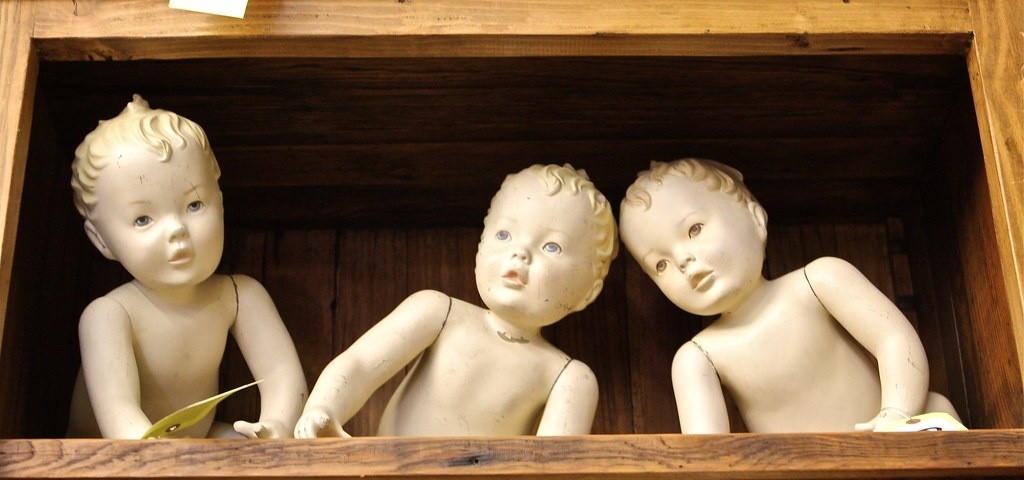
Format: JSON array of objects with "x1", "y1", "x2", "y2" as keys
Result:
[
  {"x1": 615, "y1": 152, "x2": 973, "y2": 434},
  {"x1": 292, "y1": 159, "x2": 620, "y2": 436},
  {"x1": 57, "y1": 93, "x2": 311, "y2": 441}
]
